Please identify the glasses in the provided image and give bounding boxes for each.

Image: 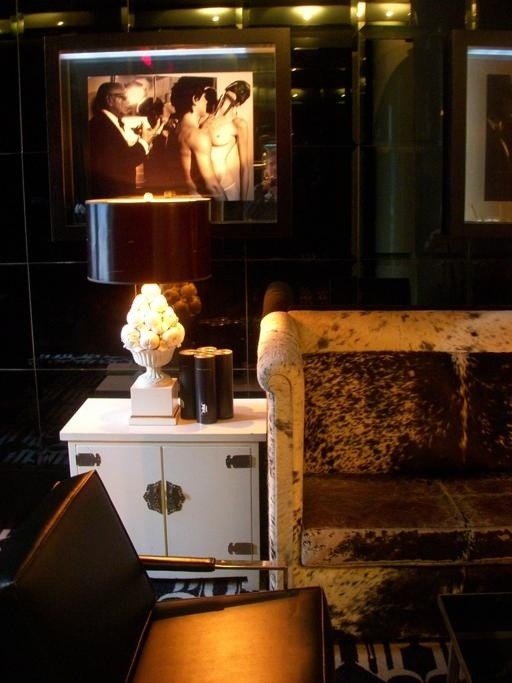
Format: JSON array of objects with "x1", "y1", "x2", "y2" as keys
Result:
[{"x1": 111, "y1": 92, "x2": 127, "y2": 99}]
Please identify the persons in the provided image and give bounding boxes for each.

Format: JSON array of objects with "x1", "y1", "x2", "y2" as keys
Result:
[
  {"x1": 205, "y1": 80, "x2": 251, "y2": 202},
  {"x1": 169, "y1": 78, "x2": 227, "y2": 204},
  {"x1": 86, "y1": 81, "x2": 165, "y2": 191}
]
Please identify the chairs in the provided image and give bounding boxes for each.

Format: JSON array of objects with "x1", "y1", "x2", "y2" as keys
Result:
[{"x1": 0, "y1": 469, "x2": 336, "y2": 683}]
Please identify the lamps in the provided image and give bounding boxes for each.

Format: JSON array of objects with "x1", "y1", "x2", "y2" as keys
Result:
[{"x1": 83, "y1": 194, "x2": 215, "y2": 286}]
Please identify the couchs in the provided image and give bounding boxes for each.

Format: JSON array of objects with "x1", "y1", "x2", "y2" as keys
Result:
[{"x1": 255, "y1": 308, "x2": 512, "y2": 647}]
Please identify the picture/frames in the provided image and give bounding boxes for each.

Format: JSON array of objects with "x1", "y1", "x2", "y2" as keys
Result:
[
  {"x1": 461, "y1": 48, "x2": 512, "y2": 225},
  {"x1": 44, "y1": 19, "x2": 293, "y2": 242}
]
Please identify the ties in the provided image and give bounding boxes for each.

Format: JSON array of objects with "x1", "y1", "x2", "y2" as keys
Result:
[{"x1": 118, "y1": 118, "x2": 124, "y2": 130}]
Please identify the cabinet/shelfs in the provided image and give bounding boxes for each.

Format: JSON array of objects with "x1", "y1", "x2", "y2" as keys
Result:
[{"x1": 60, "y1": 398, "x2": 270, "y2": 592}]
